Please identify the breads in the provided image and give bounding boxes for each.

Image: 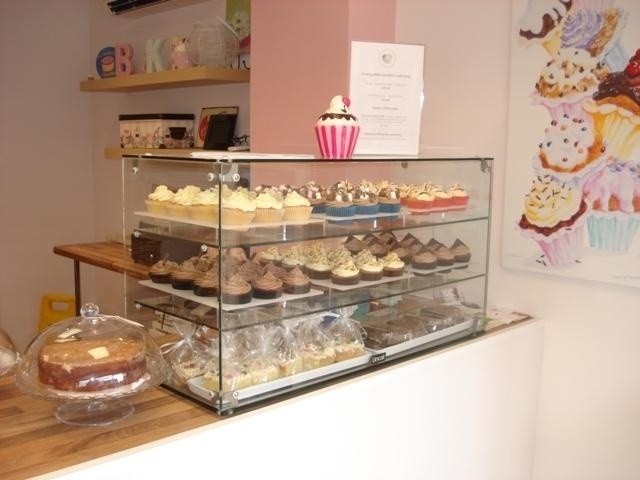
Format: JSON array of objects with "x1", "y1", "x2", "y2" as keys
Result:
[{"x1": 37, "y1": 336, "x2": 146, "y2": 392}]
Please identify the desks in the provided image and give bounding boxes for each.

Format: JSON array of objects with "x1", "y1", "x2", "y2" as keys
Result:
[{"x1": 53, "y1": 241, "x2": 151, "y2": 316}]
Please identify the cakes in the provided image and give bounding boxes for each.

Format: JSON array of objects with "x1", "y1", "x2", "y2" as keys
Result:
[
  {"x1": 517, "y1": 0, "x2": 640, "y2": 268},
  {"x1": 315, "y1": 95, "x2": 359, "y2": 159}
]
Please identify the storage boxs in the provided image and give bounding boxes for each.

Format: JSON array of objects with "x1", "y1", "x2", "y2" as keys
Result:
[{"x1": 118, "y1": 114, "x2": 194, "y2": 147}]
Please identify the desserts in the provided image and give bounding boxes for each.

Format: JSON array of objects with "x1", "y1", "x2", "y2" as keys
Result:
[{"x1": 146, "y1": 180, "x2": 471, "y2": 393}]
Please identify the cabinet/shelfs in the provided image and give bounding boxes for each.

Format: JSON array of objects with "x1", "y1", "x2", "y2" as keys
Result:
[
  {"x1": 122, "y1": 156, "x2": 494, "y2": 416},
  {"x1": 80, "y1": 0, "x2": 250, "y2": 171}
]
